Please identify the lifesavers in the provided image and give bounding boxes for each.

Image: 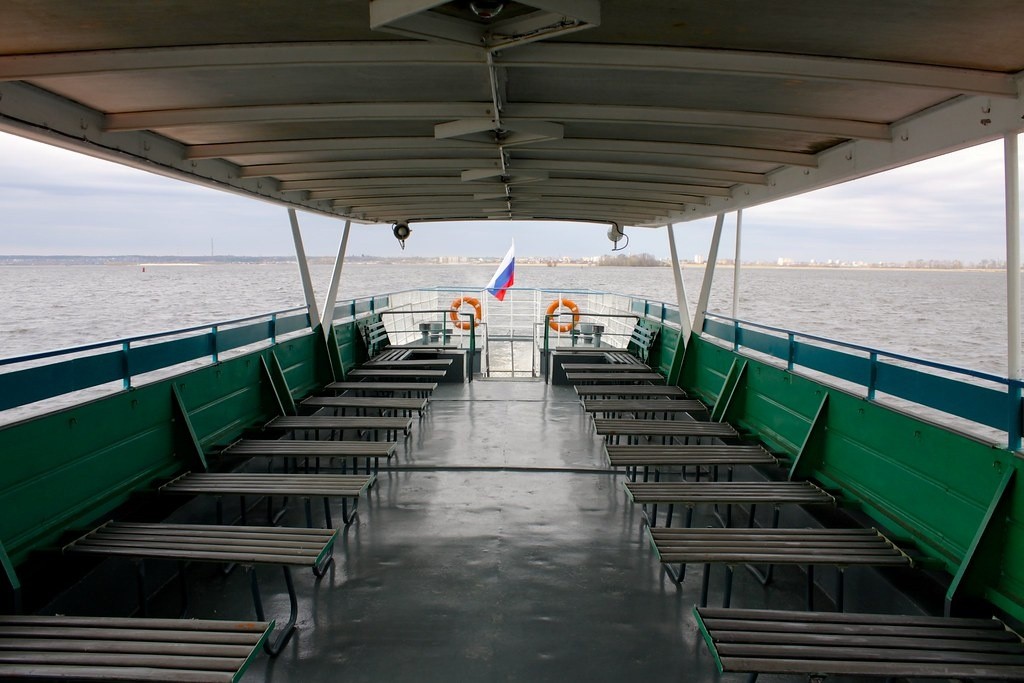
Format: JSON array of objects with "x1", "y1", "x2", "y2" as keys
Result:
[
  {"x1": 546, "y1": 298, "x2": 580, "y2": 332},
  {"x1": 450, "y1": 296, "x2": 482, "y2": 330}
]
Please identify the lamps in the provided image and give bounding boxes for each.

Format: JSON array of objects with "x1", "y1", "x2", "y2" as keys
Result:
[{"x1": 367, "y1": 0, "x2": 601, "y2": 217}]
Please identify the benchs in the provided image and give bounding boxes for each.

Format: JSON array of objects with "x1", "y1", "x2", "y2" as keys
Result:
[
  {"x1": 555, "y1": 328, "x2": 1024, "y2": 683},
  {"x1": 0, "y1": 338, "x2": 453, "y2": 683}
]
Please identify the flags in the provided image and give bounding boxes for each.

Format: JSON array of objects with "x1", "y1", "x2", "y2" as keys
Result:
[{"x1": 485, "y1": 244, "x2": 515, "y2": 302}]
